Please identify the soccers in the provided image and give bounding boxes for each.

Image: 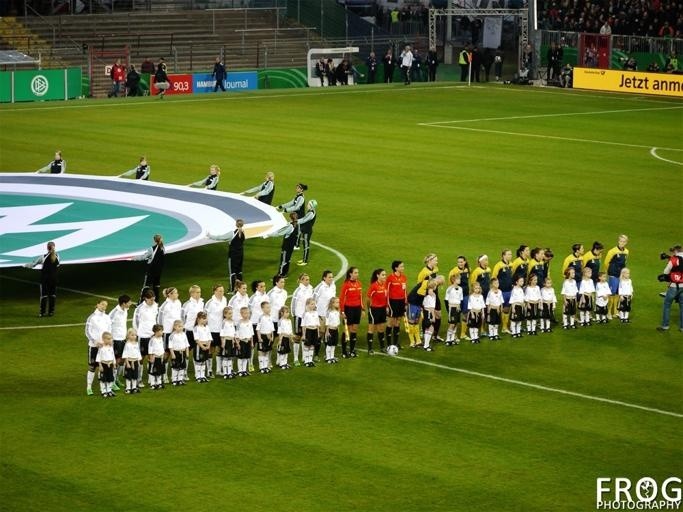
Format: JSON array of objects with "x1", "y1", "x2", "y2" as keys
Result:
[{"x1": 387, "y1": 345, "x2": 399, "y2": 357}]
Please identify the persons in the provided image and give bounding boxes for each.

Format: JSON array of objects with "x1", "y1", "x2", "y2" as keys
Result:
[
  {"x1": 81, "y1": 233, "x2": 633, "y2": 399},
  {"x1": 108, "y1": 57, "x2": 125, "y2": 97},
  {"x1": 25, "y1": 241, "x2": 60, "y2": 318},
  {"x1": 314, "y1": 0, "x2": 682, "y2": 89},
  {"x1": 205, "y1": 219, "x2": 244, "y2": 295},
  {"x1": 141, "y1": 56, "x2": 155, "y2": 75},
  {"x1": 153, "y1": 64, "x2": 170, "y2": 100},
  {"x1": 240, "y1": 171, "x2": 274, "y2": 206},
  {"x1": 117, "y1": 157, "x2": 150, "y2": 180},
  {"x1": 211, "y1": 56, "x2": 227, "y2": 92},
  {"x1": 159, "y1": 57, "x2": 167, "y2": 75},
  {"x1": 260, "y1": 212, "x2": 300, "y2": 280},
  {"x1": 655, "y1": 246, "x2": 682, "y2": 331},
  {"x1": 287, "y1": 200, "x2": 316, "y2": 267},
  {"x1": 126, "y1": 64, "x2": 140, "y2": 97},
  {"x1": 187, "y1": 165, "x2": 219, "y2": 191},
  {"x1": 276, "y1": 184, "x2": 306, "y2": 250},
  {"x1": 36, "y1": 151, "x2": 66, "y2": 173}
]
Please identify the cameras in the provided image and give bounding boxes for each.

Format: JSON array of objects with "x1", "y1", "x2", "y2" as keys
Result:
[{"x1": 661, "y1": 253, "x2": 670, "y2": 260}]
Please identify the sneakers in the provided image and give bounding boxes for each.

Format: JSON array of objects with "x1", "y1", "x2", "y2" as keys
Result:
[{"x1": 409, "y1": 314, "x2": 669, "y2": 352}]
